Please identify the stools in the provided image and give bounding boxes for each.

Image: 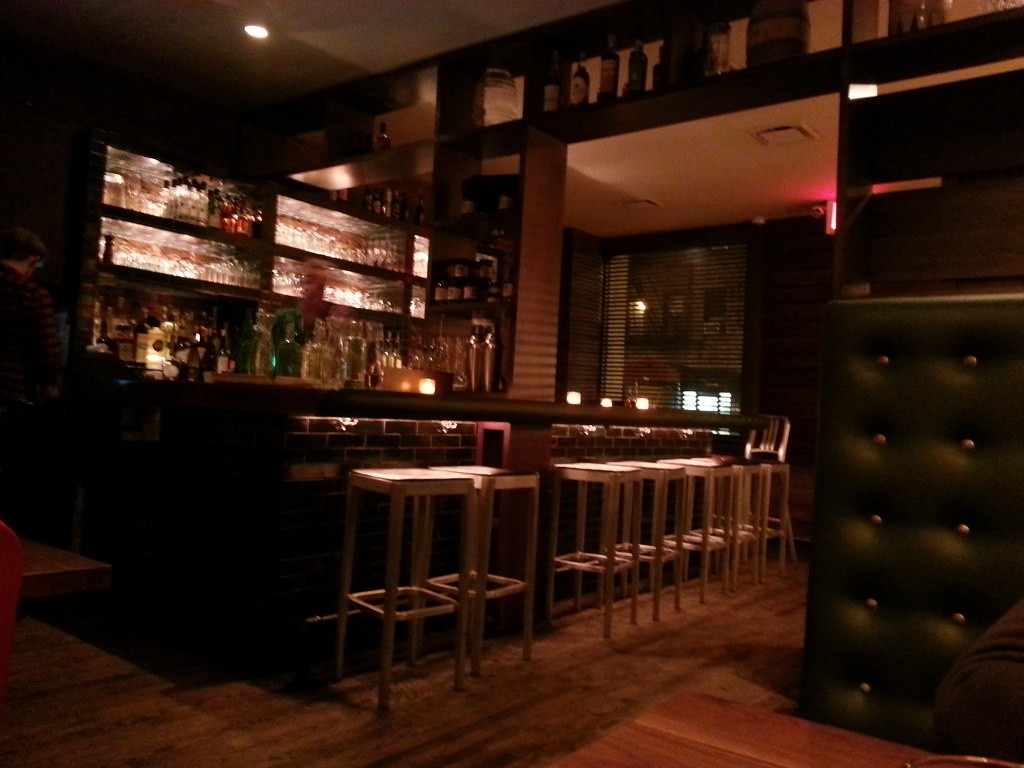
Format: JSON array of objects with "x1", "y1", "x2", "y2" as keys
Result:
[
  {"x1": 547, "y1": 416, "x2": 797, "y2": 639},
  {"x1": 336, "y1": 465, "x2": 540, "y2": 709}
]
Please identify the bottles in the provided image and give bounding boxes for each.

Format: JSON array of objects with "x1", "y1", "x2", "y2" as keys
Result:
[
  {"x1": 432, "y1": 256, "x2": 500, "y2": 300},
  {"x1": 652, "y1": 45, "x2": 667, "y2": 94},
  {"x1": 703, "y1": 16, "x2": 732, "y2": 76},
  {"x1": 627, "y1": 40, "x2": 647, "y2": 94},
  {"x1": 328, "y1": 184, "x2": 425, "y2": 225},
  {"x1": 596, "y1": 33, "x2": 620, "y2": 103},
  {"x1": 159, "y1": 178, "x2": 258, "y2": 236},
  {"x1": 80, "y1": 285, "x2": 497, "y2": 393},
  {"x1": 374, "y1": 122, "x2": 390, "y2": 150},
  {"x1": 543, "y1": 50, "x2": 560, "y2": 112},
  {"x1": 568, "y1": 50, "x2": 590, "y2": 107}
]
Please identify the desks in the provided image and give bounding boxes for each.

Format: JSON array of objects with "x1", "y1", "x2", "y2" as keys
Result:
[{"x1": 17, "y1": 540, "x2": 111, "y2": 603}]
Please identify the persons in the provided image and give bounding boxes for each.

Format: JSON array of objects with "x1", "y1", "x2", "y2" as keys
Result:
[
  {"x1": 0, "y1": 228, "x2": 65, "y2": 539},
  {"x1": 929, "y1": 595, "x2": 1024, "y2": 759}
]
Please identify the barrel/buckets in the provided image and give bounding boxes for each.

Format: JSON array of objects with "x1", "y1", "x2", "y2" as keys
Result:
[
  {"x1": 472, "y1": 68, "x2": 518, "y2": 126},
  {"x1": 746, "y1": 0, "x2": 812, "y2": 67}
]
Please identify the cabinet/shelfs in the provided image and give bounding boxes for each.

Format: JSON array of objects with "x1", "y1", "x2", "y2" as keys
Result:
[{"x1": 70, "y1": 143, "x2": 434, "y2": 326}]
